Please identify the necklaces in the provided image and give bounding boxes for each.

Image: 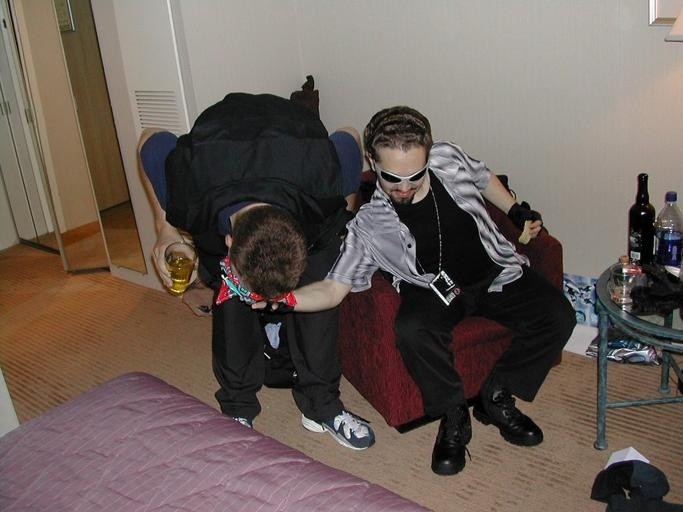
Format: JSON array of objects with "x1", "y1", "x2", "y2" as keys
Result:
[{"x1": 416, "y1": 170, "x2": 443, "y2": 276}]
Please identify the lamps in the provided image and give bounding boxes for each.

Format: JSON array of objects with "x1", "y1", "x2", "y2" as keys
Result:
[{"x1": 663, "y1": 8, "x2": 682, "y2": 43}]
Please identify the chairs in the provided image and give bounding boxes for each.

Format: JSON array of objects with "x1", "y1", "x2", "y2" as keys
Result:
[{"x1": 336, "y1": 170, "x2": 564, "y2": 429}]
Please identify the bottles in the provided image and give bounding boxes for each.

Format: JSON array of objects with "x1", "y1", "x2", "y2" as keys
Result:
[{"x1": 627, "y1": 173, "x2": 683, "y2": 269}]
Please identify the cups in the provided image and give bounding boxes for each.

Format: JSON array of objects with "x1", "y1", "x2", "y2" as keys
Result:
[
  {"x1": 163, "y1": 242, "x2": 196, "y2": 297},
  {"x1": 610, "y1": 262, "x2": 632, "y2": 305}
]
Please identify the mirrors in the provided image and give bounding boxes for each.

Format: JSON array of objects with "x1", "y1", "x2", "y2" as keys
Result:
[{"x1": 0, "y1": 1, "x2": 149, "y2": 278}]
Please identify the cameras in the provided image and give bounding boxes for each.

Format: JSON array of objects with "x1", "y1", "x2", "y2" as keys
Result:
[{"x1": 429, "y1": 270, "x2": 461, "y2": 307}]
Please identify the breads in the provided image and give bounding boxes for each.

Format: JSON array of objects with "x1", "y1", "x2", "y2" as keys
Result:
[{"x1": 519, "y1": 220, "x2": 533, "y2": 245}]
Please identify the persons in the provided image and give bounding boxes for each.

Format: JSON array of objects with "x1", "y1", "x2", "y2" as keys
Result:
[
  {"x1": 135, "y1": 73, "x2": 377, "y2": 452},
  {"x1": 238, "y1": 104, "x2": 579, "y2": 476}
]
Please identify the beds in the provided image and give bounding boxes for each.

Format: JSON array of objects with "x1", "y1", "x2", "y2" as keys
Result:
[{"x1": 1, "y1": 369, "x2": 446, "y2": 512}]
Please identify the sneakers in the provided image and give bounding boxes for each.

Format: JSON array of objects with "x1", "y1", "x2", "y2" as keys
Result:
[{"x1": 302, "y1": 409, "x2": 373, "y2": 450}]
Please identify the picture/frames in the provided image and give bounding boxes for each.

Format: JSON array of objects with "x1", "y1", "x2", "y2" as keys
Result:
[{"x1": 648, "y1": 0, "x2": 683, "y2": 26}]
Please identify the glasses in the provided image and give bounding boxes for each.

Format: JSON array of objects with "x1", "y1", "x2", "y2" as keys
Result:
[{"x1": 375, "y1": 160, "x2": 429, "y2": 184}]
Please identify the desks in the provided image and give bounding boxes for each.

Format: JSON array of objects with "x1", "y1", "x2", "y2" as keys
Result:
[{"x1": 591, "y1": 257, "x2": 683, "y2": 451}]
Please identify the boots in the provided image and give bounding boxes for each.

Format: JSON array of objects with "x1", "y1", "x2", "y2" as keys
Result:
[
  {"x1": 431, "y1": 399, "x2": 472, "y2": 475},
  {"x1": 473, "y1": 377, "x2": 543, "y2": 446}
]
